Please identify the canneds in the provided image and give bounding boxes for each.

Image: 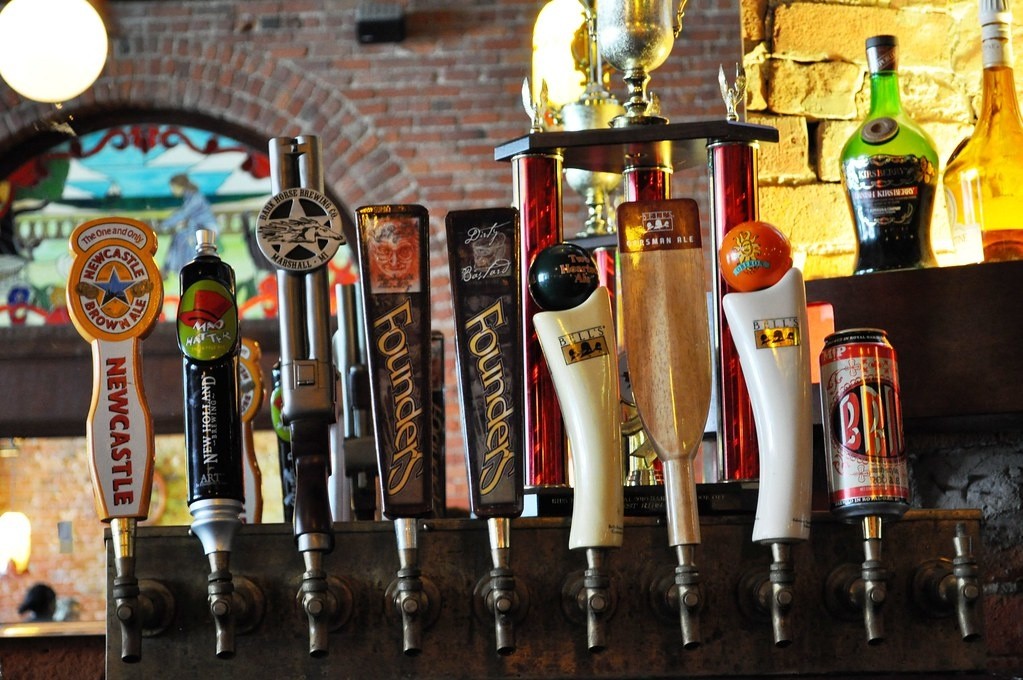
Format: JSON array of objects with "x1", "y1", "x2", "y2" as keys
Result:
[{"x1": 819, "y1": 328, "x2": 911, "y2": 525}]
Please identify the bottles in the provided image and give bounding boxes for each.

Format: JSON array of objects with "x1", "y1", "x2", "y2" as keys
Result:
[
  {"x1": 840, "y1": 35, "x2": 937, "y2": 276},
  {"x1": 943, "y1": 0, "x2": 1023, "y2": 261}
]
